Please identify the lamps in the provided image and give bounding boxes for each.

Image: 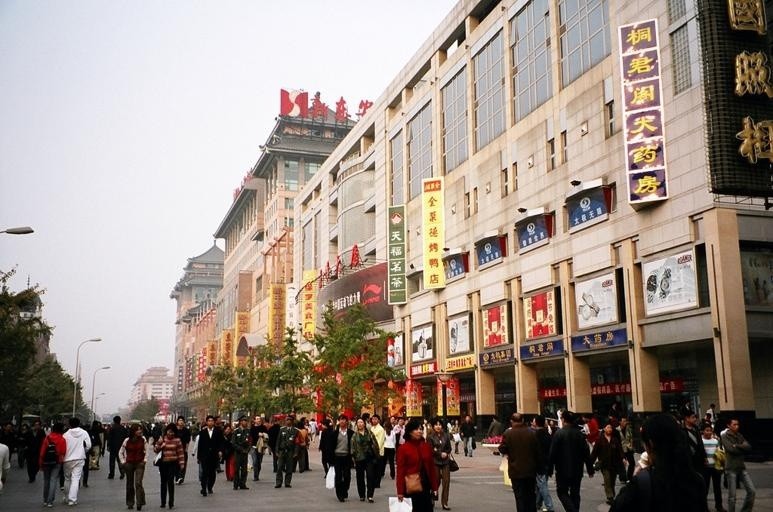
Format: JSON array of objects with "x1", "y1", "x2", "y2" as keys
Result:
[
  {"x1": 628, "y1": 341, "x2": 633, "y2": 349},
  {"x1": 474, "y1": 364, "x2": 478, "y2": 370},
  {"x1": 443, "y1": 247, "x2": 449, "y2": 251},
  {"x1": 441, "y1": 369, "x2": 443, "y2": 372},
  {"x1": 410, "y1": 264, "x2": 413, "y2": 268},
  {"x1": 563, "y1": 351, "x2": 568, "y2": 358},
  {"x1": 713, "y1": 328, "x2": 720, "y2": 337},
  {"x1": 517, "y1": 208, "x2": 527, "y2": 213},
  {"x1": 571, "y1": 180, "x2": 581, "y2": 186},
  {"x1": 514, "y1": 358, "x2": 518, "y2": 364}
]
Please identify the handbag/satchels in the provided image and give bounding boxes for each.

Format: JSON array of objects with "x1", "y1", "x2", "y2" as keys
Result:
[
  {"x1": 325, "y1": 466, "x2": 336, "y2": 489},
  {"x1": 152, "y1": 451, "x2": 162, "y2": 465},
  {"x1": 404, "y1": 473, "x2": 422, "y2": 493},
  {"x1": 387, "y1": 496, "x2": 413, "y2": 512},
  {"x1": 89, "y1": 455, "x2": 100, "y2": 470},
  {"x1": 471, "y1": 439, "x2": 477, "y2": 450},
  {"x1": 448, "y1": 453, "x2": 459, "y2": 472}
]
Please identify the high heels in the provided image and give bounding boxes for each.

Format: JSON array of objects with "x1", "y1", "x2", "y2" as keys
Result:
[{"x1": 433, "y1": 490, "x2": 451, "y2": 510}]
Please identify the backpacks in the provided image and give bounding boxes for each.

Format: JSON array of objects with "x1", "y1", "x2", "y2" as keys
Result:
[
  {"x1": 43, "y1": 436, "x2": 57, "y2": 470},
  {"x1": 713, "y1": 432, "x2": 728, "y2": 471}
]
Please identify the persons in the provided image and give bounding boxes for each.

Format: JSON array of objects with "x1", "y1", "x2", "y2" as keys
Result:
[
  {"x1": 192, "y1": 415, "x2": 320, "y2": 497},
  {"x1": 319, "y1": 412, "x2": 453, "y2": 512},
  {"x1": 498, "y1": 403, "x2": 756, "y2": 512},
  {"x1": 1, "y1": 414, "x2": 191, "y2": 510},
  {"x1": 451, "y1": 416, "x2": 501, "y2": 457}
]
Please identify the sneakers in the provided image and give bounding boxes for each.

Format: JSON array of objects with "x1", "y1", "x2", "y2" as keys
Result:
[
  {"x1": 127, "y1": 502, "x2": 176, "y2": 511},
  {"x1": 455, "y1": 450, "x2": 473, "y2": 458},
  {"x1": 587, "y1": 474, "x2": 625, "y2": 505},
  {"x1": 175, "y1": 467, "x2": 314, "y2": 497},
  {"x1": 108, "y1": 473, "x2": 125, "y2": 480},
  {"x1": 43, "y1": 497, "x2": 78, "y2": 508},
  {"x1": 340, "y1": 494, "x2": 375, "y2": 503},
  {"x1": 541, "y1": 505, "x2": 553, "y2": 511}
]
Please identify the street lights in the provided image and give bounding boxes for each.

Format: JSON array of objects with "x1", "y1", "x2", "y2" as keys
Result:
[
  {"x1": 2, "y1": 224, "x2": 37, "y2": 243},
  {"x1": 434, "y1": 371, "x2": 455, "y2": 433},
  {"x1": 70, "y1": 336, "x2": 112, "y2": 431}
]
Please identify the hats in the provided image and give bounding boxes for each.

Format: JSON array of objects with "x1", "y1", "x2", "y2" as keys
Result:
[
  {"x1": 236, "y1": 414, "x2": 248, "y2": 421},
  {"x1": 286, "y1": 414, "x2": 295, "y2": 420}
]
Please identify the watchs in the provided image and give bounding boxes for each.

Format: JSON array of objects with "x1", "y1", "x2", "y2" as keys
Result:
[
  {"x1": 646, "y1": 269, "x2": 660, "y2": 303},
  {"x1": 658, "y1": 268, "x2": 672, "y2": 299},
  {"x1": 578, "y1": 292, "x2": 600, "y2": 321},
  {"x1": 450, "y1": 322, "x2": 458, "y2": 352}
]
